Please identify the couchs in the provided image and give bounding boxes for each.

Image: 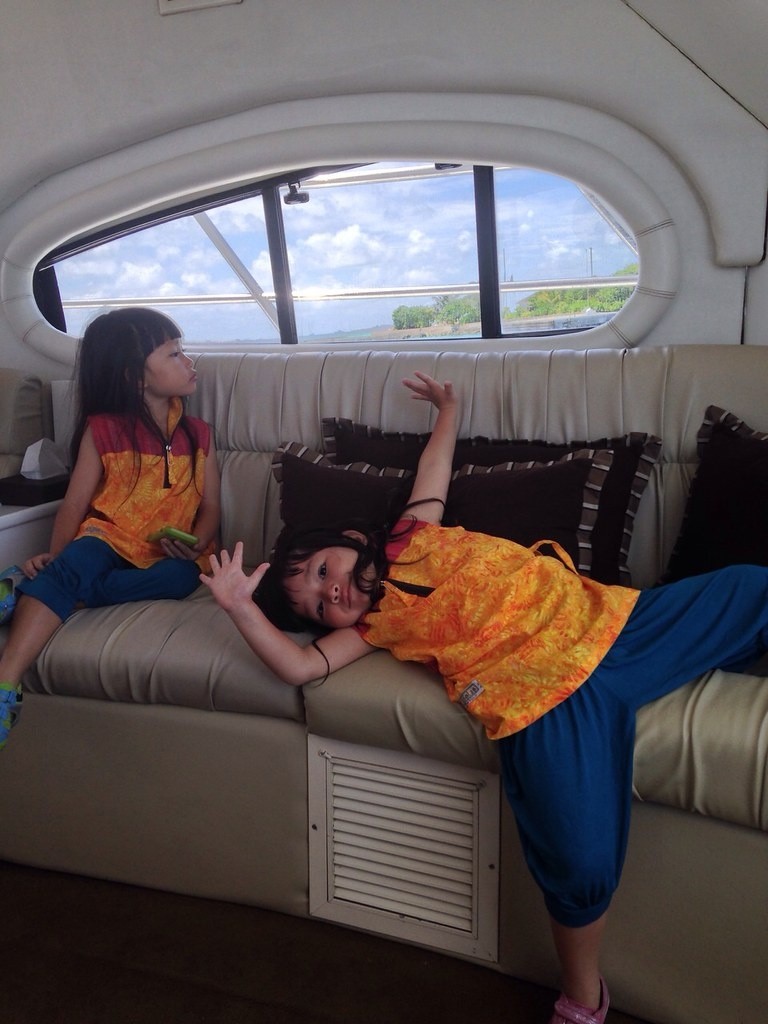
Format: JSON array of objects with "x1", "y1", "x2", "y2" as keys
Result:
[{"x1": 0, "y1": 346, "x2": 768, "y2": 1024}]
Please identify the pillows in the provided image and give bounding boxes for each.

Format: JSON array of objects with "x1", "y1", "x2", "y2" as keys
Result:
[
  {"x1": 668, "y1": 404, "x2": 768, "y2": 577},
  {"x1": 265, "y1": 416, "x2": 660, "y2": 587}
]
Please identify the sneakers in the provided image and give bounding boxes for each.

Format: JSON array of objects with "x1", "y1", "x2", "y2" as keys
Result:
[
  {"x1": 552, "y1": 975, "x2": 610, "y2": 1024},
  {"x1": 0, "y1": 565, "x2": 27, "y2": 623},
  {"x1": 0, "y1": 682, "x2": 23, "y2": 750}
]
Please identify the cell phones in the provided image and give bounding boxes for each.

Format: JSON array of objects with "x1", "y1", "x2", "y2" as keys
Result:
[{"x1": 147, "y1": 525, "x2": 199, "y2": 549}]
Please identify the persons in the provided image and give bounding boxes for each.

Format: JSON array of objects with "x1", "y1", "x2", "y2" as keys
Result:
[
  {"x1": 198, "y1": 372, "x2": 768, "y2": 1024},
  {"x1": 0, "y1": 308, "x2": 222, "y2": 745}
]
